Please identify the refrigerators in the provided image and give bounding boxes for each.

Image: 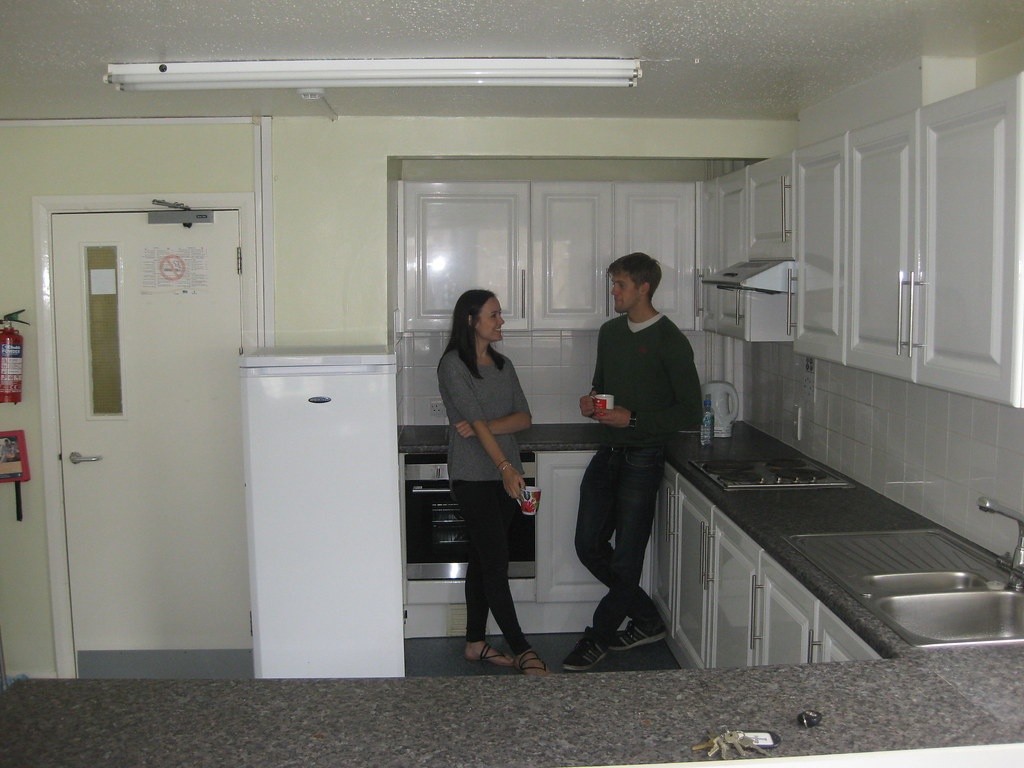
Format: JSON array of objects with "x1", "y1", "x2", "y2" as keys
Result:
[{"x1": 237, "y1": 348, "x2": 408, "y2": 678}]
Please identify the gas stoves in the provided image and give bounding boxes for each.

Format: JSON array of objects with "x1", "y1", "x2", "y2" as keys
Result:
[{"x1": 686, "y1": 454, "x2": 853, "y2": 490}]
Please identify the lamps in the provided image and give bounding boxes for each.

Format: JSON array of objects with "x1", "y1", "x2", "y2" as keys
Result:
[{"x1": 100, "y1": 57, "x2": 643, "y2": 93}]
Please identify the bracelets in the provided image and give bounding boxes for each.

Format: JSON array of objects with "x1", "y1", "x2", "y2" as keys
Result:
[
  {"x1": 629, "y1": 412, "x2": 636, "y2": 428},
  {"x1": 497, "y1": 459, "x2": 507, "y2": 469},
  {"x1": 501, "y1": 463, "x2": 511, "y2": 471}
]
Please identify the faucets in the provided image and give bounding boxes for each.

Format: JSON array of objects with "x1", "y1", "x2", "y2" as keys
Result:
[{"x1": 976, "y1": 494, "x2": 1024, "y2": 575}]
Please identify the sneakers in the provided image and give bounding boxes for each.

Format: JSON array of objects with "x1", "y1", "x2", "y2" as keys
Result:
[
  {"x1": 609, "y1": 621, "x2": 667, "y2": 650},
  {"x1": 562, "y1": 626, "x2": 609, "y2": 671}
]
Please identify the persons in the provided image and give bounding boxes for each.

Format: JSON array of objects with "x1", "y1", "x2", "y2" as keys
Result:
[
  {"x1": 561, "y1": 251, "x2": 703, "y2": 671},
  {"x1": 438, "y1": 289, "x2": 553, "y2": 675}
]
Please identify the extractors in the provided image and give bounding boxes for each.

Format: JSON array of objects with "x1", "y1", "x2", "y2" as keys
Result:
[{"x1": 701, "y1": 258, "x2": 798, "y2": 294}]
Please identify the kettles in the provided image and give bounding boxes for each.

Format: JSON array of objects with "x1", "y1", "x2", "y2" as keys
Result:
[{"x1": 700, "y1": 380, "x2": 739, "y2": 438}]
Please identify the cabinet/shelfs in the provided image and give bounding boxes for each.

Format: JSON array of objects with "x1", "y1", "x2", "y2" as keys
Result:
[
  {"x1": 392, "y1": 70, "x2": 1024, "y2": 410},
  {"x1": 530, "y1": 452, "x2": 882, "y2": 672}
]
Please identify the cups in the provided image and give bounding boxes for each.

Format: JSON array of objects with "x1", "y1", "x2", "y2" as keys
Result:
[
  {"x1": 589, "y1": 394, "x2": 615, "y2": 417},
  {"x1": 516, "y1": 486, "x2": 541, "y2": 515}
]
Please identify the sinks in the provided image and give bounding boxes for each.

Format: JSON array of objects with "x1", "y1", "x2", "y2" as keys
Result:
[
  {"x1": 876, "y1": 590, "x2": 1023, "y2": 642},
  {"x1": 867, "y1": 572, "x2": 992, "y2": 596}
]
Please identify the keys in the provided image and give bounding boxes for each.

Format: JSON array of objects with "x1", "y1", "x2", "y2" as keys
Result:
[
  {"x1": 798, "y1": 710, "x2": 822, "y2": 726},
  {"x1": 691, "y1": 730, "x2": 771, "y2": 760}
]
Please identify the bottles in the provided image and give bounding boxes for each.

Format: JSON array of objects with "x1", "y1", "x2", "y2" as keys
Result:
[{"x1": 699, "y1": 400, "x2": 714, "y2": 447}]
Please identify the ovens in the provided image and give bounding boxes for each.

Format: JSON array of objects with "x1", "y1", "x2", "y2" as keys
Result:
[{"x1": 401, "y1": 449, "x2": 541, "y2": 580}]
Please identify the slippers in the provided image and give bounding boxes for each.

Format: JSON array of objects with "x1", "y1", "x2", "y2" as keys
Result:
[
  {"x1": 465, "y1": 643, "x2": 514, "y2": 666},
  {"x1": 514, "y1": 651, "x2": 548, "y2": 675}
]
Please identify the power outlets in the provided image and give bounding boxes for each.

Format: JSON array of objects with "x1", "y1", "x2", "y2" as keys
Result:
[{"x1": 802, "y1": 358, "x2": 817, "y2": 402}]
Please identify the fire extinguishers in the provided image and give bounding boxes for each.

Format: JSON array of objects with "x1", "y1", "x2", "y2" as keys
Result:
[{"x1": 0, "y1": 309, "x2": 30, "y2": 405}]
